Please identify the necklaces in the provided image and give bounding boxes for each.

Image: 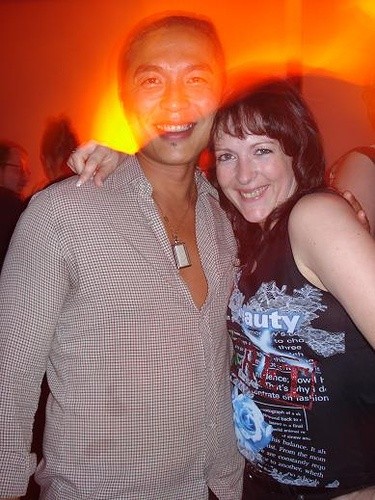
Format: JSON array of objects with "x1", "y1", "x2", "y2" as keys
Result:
[{"x1": 141, "y1": 160, "x2": 198, "y2": 269}]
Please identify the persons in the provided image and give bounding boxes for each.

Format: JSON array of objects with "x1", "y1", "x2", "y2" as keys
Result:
[
  {"x1": 0, "y1": 140, "x2": 35, "y2": 268},
  {"x1": 0, "y1": 12, "x2": 247, "y2": 500},
  {"x1": 26, "y1": 116, "x2": 83, "y2": 194},
  {"x1": 209, "y1": 78, "x2": 374, "y2": 500}
]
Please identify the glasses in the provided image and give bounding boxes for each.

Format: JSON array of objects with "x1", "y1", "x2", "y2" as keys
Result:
[{"x1": 1, "y1": 161, "x2": 30, "y2": 176}]
